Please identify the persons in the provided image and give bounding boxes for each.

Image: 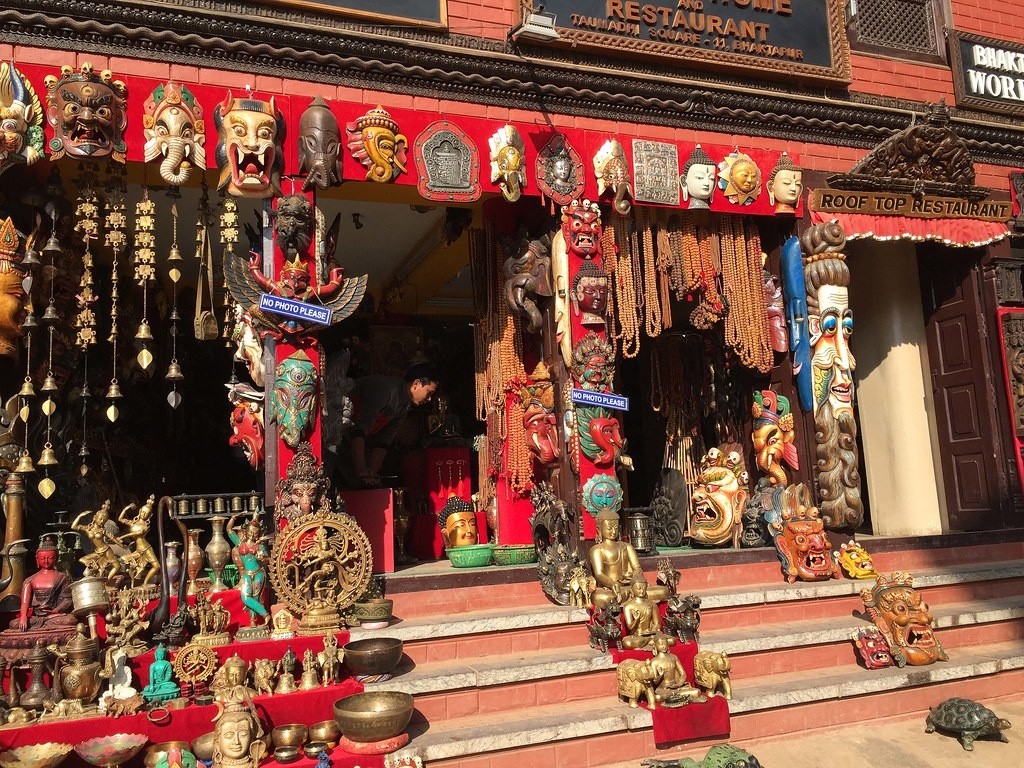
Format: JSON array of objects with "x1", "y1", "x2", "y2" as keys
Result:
[{"x1": 331, "y1": 362, "x2": 439, "y2": 490}]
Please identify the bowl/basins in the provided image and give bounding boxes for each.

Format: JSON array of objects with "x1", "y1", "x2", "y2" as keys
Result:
[
  {"x1": 491, "y1": 544, "x2": 538, "y2": 565},
  {"x1": 445, "y1": 544, "x2": 499, "y2": 567},
  {"x1": 255, "y1": 719, "x2": 339, "y2": 762},
  {"x1": 143, "y1": 730, "x2": 215, "y2": 768},
  {"x1": 354, "y1": 598, "x2": 393, "y2": 622},
  {"x1": 333, "y1": 690, "x2": 415, "y2": 742},
  {"x1": 341, "y1": 637, "x2": 403, "y2": 675}
]
